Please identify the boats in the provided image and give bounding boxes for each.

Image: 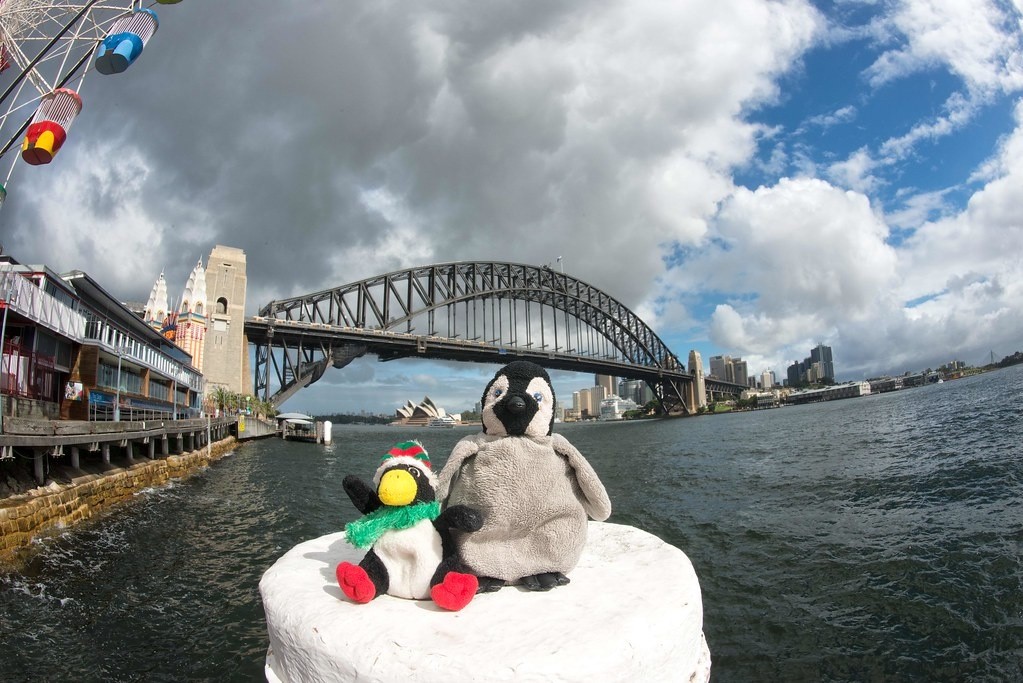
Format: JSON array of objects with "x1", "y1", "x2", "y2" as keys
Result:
[
  {"x1": 428, "y1": 416, "x2": 457, "y2": 429},
  {"x1": 275, "y1": 412, "x2": 324, "y2": 444}
]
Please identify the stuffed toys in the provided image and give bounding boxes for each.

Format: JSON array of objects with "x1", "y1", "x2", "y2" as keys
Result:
[
  {"x1": 336, "y1": 438, "x2": 484, "y2": 611},
  {"x1": 437, "y1": 362, "x2": 611, "y2": 595}
]
